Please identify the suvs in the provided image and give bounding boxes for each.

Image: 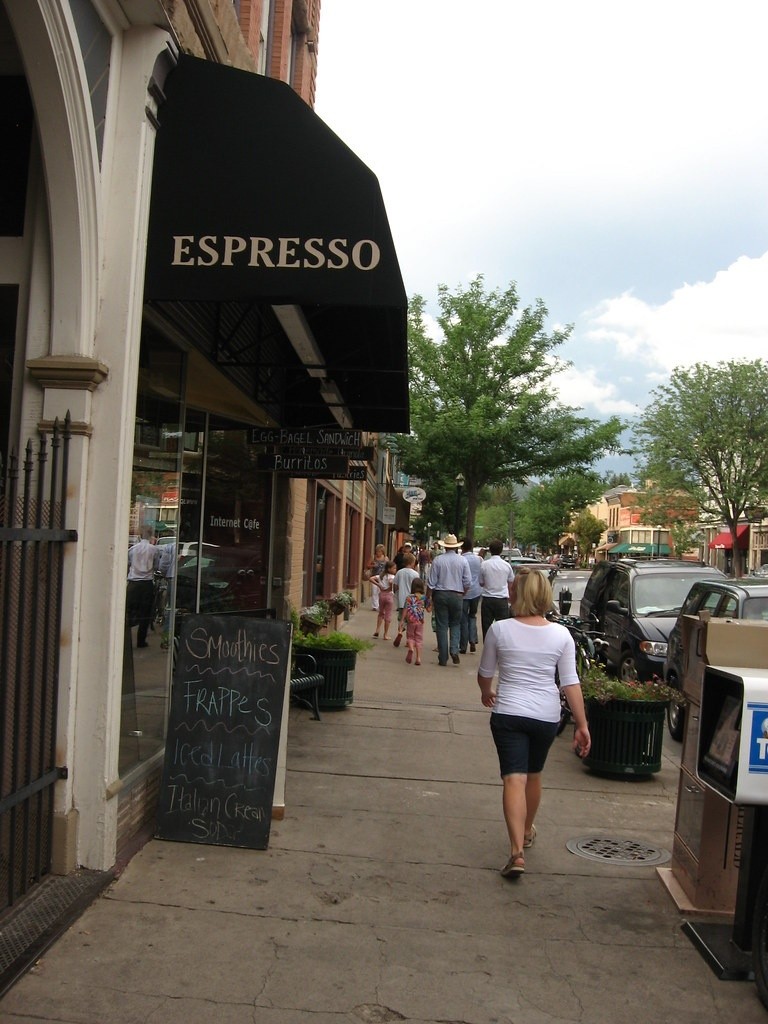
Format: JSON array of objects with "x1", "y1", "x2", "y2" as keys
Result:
[{"x1": 579, "y1": 553, "x2": 732, "y2": 690}]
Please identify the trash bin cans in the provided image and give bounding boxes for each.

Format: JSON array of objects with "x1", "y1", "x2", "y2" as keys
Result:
[{"x1": 654, "y1": 607, "x2": 768, "y2": 917}]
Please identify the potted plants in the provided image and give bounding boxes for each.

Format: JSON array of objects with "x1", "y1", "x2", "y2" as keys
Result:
[
  {"x1": 299, "y1": 600, "x2": 331, "y2": 638},
  {"x1": 291, "y1": 607, "x2": 378, "y2": 708},
  {"x1": 330, "y1": 592, "x2": 357, "y2": 615}
]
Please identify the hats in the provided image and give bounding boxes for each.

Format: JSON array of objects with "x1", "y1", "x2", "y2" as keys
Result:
[
  {"x1": 438, "y1": 533, "x2": 464, "y2": 548},
  {"x1": 403, "y1": 542, "x2": 413, "y2": 553}
]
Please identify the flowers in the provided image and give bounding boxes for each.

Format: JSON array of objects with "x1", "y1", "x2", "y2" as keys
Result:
[{"x1": 579, "y1": 657, "x2": 686, "y2": 706}]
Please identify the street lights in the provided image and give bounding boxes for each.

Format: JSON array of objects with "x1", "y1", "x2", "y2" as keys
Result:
[{"x1": 453, "y1": 472, "x2": 466, "y2": 542}]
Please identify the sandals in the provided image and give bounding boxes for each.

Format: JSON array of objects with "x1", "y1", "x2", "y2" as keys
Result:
[
  {"x1": 500, "y1": 853, "x2": 528, "y2": 878},
  {"x1": 524, "y1": 825, "x2": 538, "y2": 848}
]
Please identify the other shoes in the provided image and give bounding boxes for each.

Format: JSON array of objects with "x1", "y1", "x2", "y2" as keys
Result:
[
  {"x1": 415, "y1": 658, "x2": 422, "y2": 666},
  {"x1": 469, "y1": 644, "x2": 477, "y2": 654},
  {"x1": 136, "y1": 640, "x2": 150, "y2": 649},
  {"x1": 405, "y1": 649, "x2": 414, "y2": 664},
  {"x1": 432, "y1": 647, "x2": 440, "y2": 652},
  {"x1": 160, "y1": 639, "x2": 169, "y2": 649},
  {"x1": 458, "y1": 649, "x2": 467, "y2": 655},
  {"x1": 393, "y1": 633, "x2": 403, "y2": 648},
  {"x1": 450, "y1": 653, "x2": 461, "y2": 665},
  {"x1": 437, "y1": 661, "x2": 448, "y2": 666},
  {"x1": 372, "y1": 633, "x2": 380, "y2": 638}
]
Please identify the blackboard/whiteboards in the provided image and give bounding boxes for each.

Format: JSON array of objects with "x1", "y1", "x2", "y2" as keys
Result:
[{"x1": 153, "y1": 617, "x2": 295, "y2": 848}]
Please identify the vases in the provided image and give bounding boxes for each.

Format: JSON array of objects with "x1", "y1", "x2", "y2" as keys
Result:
[{"x1": 582, "y1": 700, "x2": 670, "y2": 776}]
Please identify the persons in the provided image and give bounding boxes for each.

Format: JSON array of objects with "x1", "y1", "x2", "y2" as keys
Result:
[
  {"x1": 367, "y1": 530, "x2": 514, "y2": 666},
  {"x1": 127, "y1": 525, "x2": 177, "y2": 648},
  {"x1": 477, "y1": 567, "x2": 591, "y2": 879}
]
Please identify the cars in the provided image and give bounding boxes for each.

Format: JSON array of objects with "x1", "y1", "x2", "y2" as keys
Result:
[
  {"x1": 754, "y1": 563, "x2": 768, "y2": 579},
  {"x1": 128, "y1": 535, "x2": 142, "y2": 550},
  {"x1": 155, "y1": 536, "x2": 178, "y2": 557},
  {"x1": 473, "y1": 546, "x2": 592, "y2": 637},
  {"x1": 663, "y1": 578, "x2": 768, "y2": 741},
  {"x1": 178, "y1": 540, "x2": 227, "y2": 589}
]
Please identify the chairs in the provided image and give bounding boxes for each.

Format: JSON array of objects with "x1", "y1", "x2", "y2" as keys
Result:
[{"x1": 291, "y1": 653, "x2": 325, "y2": 720}]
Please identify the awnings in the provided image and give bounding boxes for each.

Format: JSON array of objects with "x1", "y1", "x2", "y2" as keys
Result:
[
  {"x1": 596, "y1": 542, "x2": 671, "y2": 554},
  {"x1": 708, "y1": 525, "x2": 749, "y2": 550},
  {"x1": 138, "y1": 52, "x2": 412, "y2": 434},
  {"x1": 386, "y1": 485, "x2": 411, "y2": 535}
]
